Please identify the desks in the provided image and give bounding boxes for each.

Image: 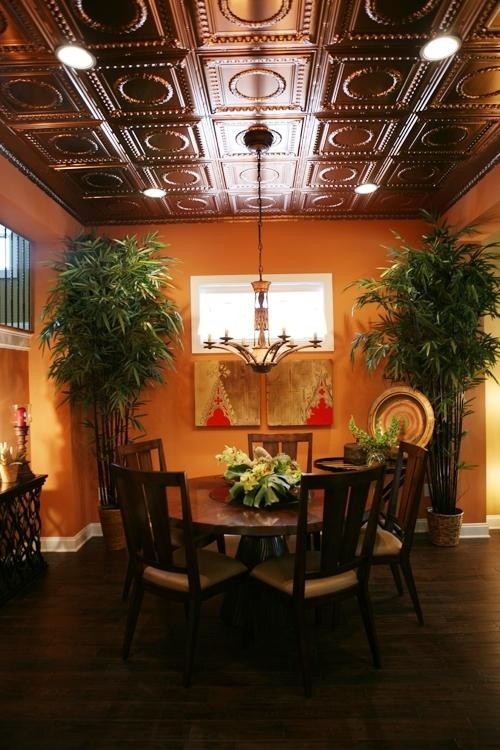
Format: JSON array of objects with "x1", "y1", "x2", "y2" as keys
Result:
[
  {"x1": 314, "y1": 454, "x2": 405, "y2": 539},
  {"x1": 160, "y1": 470, "x2": 388, "y2": 633},
  {"x1": 1, "y1": 472, "x2": 48, "y2": 599}
]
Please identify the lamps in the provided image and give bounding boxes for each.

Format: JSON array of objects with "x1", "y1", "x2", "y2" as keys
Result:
[{"x1": 199, "y1": 125, "x2": 322, "y2": 375}]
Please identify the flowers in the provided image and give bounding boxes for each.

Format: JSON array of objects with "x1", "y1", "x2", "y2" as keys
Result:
[{"x1": 214, "y1": 440, "x2": 306, "y2": 509}]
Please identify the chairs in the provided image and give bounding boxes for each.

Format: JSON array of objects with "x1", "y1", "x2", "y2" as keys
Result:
[
  {"x1": 244, "y1": 430, "x2": 314, "y2": 474},
  {"x1": 352, "y1": 438, "x2": 431, "y2": 625},
  {"x1": 112, "y1": 434, "x2": 227, "y2": 599},
  {"x1": 107, "y1": 463, "x2": 244, "y2": 685},
  {"x1": 252, "y1": 460, "x2": 393, "y2": 701}
]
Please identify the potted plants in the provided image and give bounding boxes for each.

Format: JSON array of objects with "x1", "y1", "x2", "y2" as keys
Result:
[
  {"x1": 47, "y1": 226, "x2": 184, "y2": 551},
  {"x1": 339, "y1": 208, "x2": 499, "y2": 550},
  {"x1": 0, "y1": 439, "x2": 29, "y2": 483},
  {"x1": 347, "y1": 413, "x2": 401, "y2": 468}
]
